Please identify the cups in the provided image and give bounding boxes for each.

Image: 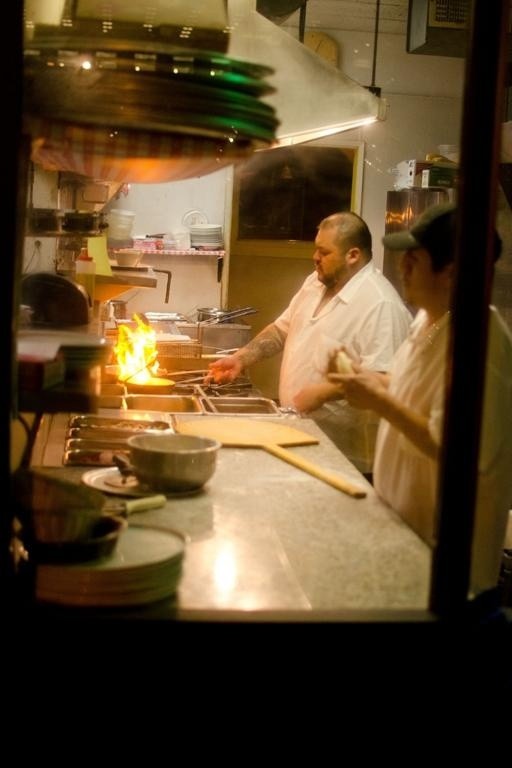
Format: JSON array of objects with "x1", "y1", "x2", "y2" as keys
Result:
[{"x1": 111, "y1": 247, "x2": 149, "y2": 272}]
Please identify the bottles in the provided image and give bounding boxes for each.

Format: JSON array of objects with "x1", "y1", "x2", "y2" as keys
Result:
[{"x1": 57, "y1": 247, "x2": 95, "y2": 320}]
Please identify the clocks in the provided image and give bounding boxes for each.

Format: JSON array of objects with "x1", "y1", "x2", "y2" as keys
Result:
[{"x1": 299, "y1": 32, "x2": 338, "y2": 70}]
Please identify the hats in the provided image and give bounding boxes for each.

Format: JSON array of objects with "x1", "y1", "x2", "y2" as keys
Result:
[{"x1": 379, "y1": 201, "x2": 502, "y2": 263}]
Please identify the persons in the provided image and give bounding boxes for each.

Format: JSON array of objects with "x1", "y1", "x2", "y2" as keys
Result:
[
  {"x1": 317, "y1": 198, "x2": 511, "y2": 607},
  {"x1": 203, "y1": 212, "x2": 411, "y2": 487}
]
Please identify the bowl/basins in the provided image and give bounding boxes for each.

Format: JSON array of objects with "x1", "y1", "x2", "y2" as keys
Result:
[
  {"x1": 197, "y1": 306, "x2": 236, "y2": 324},
  {"x1": 106, "y1": 208, "x2": 136, "y2": 240},
  {"x1": 102, "y1": 299, "x2": 128, "y2": 321},
  {"x1": 17, "y1": 510, "x2": 128, "y2": 566},
  {"x1": 125, "y1": 432, "x2": 222, "y2": 494}
]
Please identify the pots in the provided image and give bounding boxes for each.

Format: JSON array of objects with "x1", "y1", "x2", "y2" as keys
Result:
[{"x1": 102, "y1": 363, "x2": 214, "y2": 395}]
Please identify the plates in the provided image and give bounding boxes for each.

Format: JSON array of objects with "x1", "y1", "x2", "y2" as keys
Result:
[
  {"x1": 81, "y1": 465, "x2": 156, "y2": 497},
  {"x1": 181, "y1": 208, "x2": 224, "y2": 252},
  {"x1": 34, "y1": 523, "x2": 190, "y2": 606}
]
